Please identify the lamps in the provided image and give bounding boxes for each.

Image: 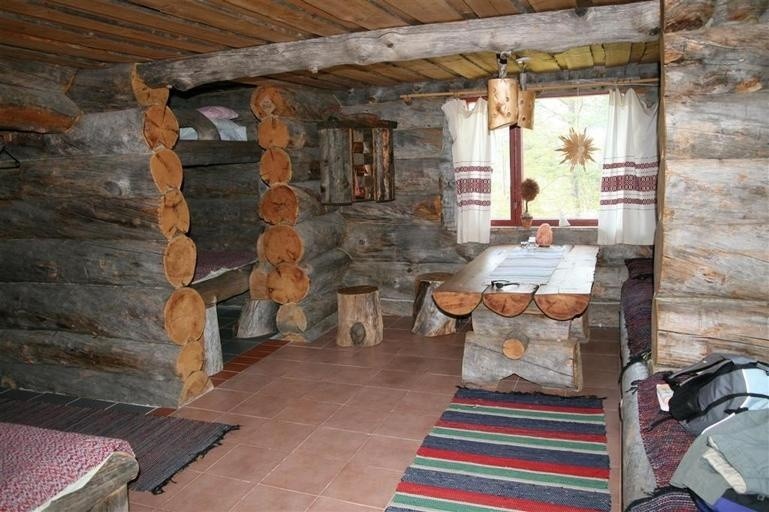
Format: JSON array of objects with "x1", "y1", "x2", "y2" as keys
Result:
[{"x1": 486, "y1": 53, "x2": 537, "y2": 131}]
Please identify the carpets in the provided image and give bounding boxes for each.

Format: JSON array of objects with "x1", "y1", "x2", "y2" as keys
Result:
[
  {"x1": 382, "y1": 383, "x2": 613, "y2": 510},
  {"x1": 1, "y1": 378, "x2": 241, "y2": 495}
]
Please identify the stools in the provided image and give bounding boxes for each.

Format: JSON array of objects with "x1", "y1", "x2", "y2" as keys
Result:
[{"x1": 334, "y1": 271, "x2": 457, "y2": 352}]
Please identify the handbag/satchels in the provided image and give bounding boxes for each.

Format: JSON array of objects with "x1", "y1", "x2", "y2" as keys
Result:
[{"x1": 648, "y1": 354, "x2": 769, "y2": 435}]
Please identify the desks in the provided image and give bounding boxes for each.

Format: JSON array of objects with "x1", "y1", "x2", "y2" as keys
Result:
[{"x1": 432, "y1": 245, "x2": 601, "y2": 394}]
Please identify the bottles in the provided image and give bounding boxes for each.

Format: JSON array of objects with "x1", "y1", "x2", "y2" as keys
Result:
[{"x1": 527, "y1": 236, "x2": 537, "y2": 254}]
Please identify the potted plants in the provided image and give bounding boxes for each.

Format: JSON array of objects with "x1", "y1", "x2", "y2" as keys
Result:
[{"x1": 517, "y1": 176, "x2": 540, "y2": 228}]
